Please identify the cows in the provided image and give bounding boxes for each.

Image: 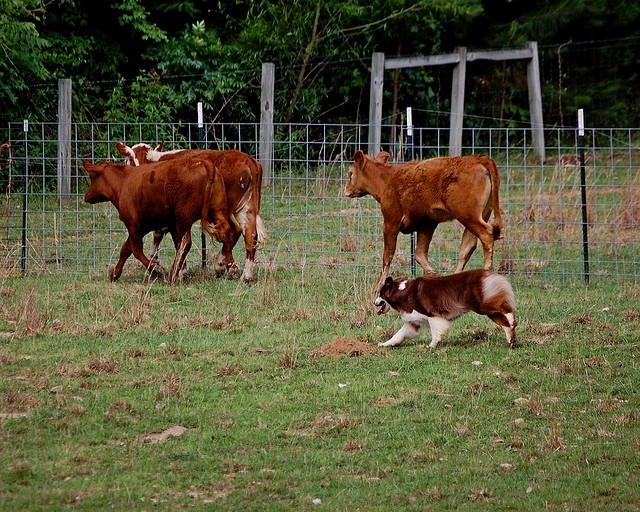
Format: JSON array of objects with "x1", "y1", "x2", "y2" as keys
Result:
[
  {"x1": 81, "y1": 156, "x2": 240, "y2": 285},
  {"x1": 116, "y1": 140, "x2": 271, "y2": 285},
  {"x1": 344, "y1": 150, "x2": 504, "y2": 293}
]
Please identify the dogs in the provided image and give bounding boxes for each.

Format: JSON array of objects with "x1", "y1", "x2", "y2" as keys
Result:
[{"x1": 372, "y1": 268, "x2": 523, "y2": 351}]
